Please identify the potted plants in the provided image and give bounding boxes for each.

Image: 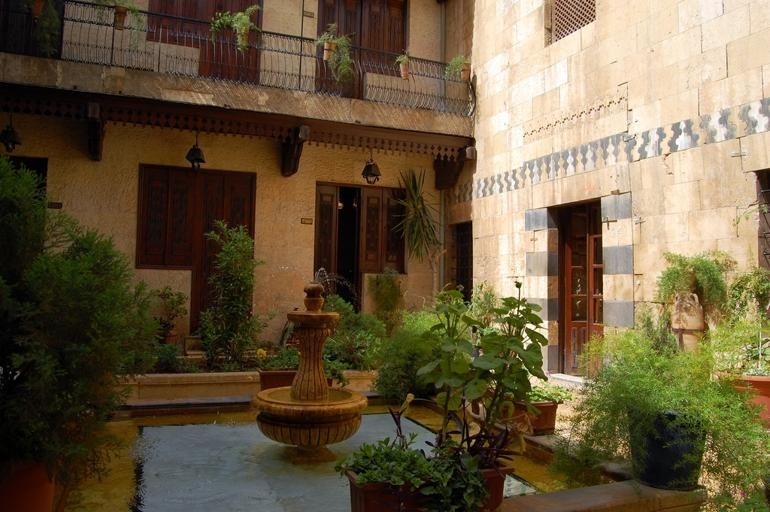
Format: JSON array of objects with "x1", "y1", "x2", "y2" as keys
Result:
[
  {"x1": 394, "y1": 53, "x2": 409, "y2": 80},
  {"x1": 1, "y1": 166, "x2": 166, "y2": 512},
  {"x1": 95, "y1": 0, "x2": 146, "y2": 48},
  {"x1": 257, "y1": 344, "x2": 351, "y2": 391},
  {"x1": 337, "y1": 164, "x2": 768, "y2": 512},
  {"x1": 316, "y1": 30, "x2": 354, "y2": 78},
  {"x1": 208, "y1": 0, "x2": 260, "y2": 55},
  {"x1": 443, "y1": 54, "x2": 471, "y2": 84},
  {"x1": 22, "y1": 0, "x2": 61, "y2": 56}
]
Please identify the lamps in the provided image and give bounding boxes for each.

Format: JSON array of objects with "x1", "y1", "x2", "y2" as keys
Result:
[
  {"x1": 185, "y1": 129, "x2": 206, "y2": 170},
  {"x1": 362, "y1": 146, "x2": 381, "y2": 184},
  {"x1": 1, "y1": 109, "x2": 22, "y2": 154}
]
